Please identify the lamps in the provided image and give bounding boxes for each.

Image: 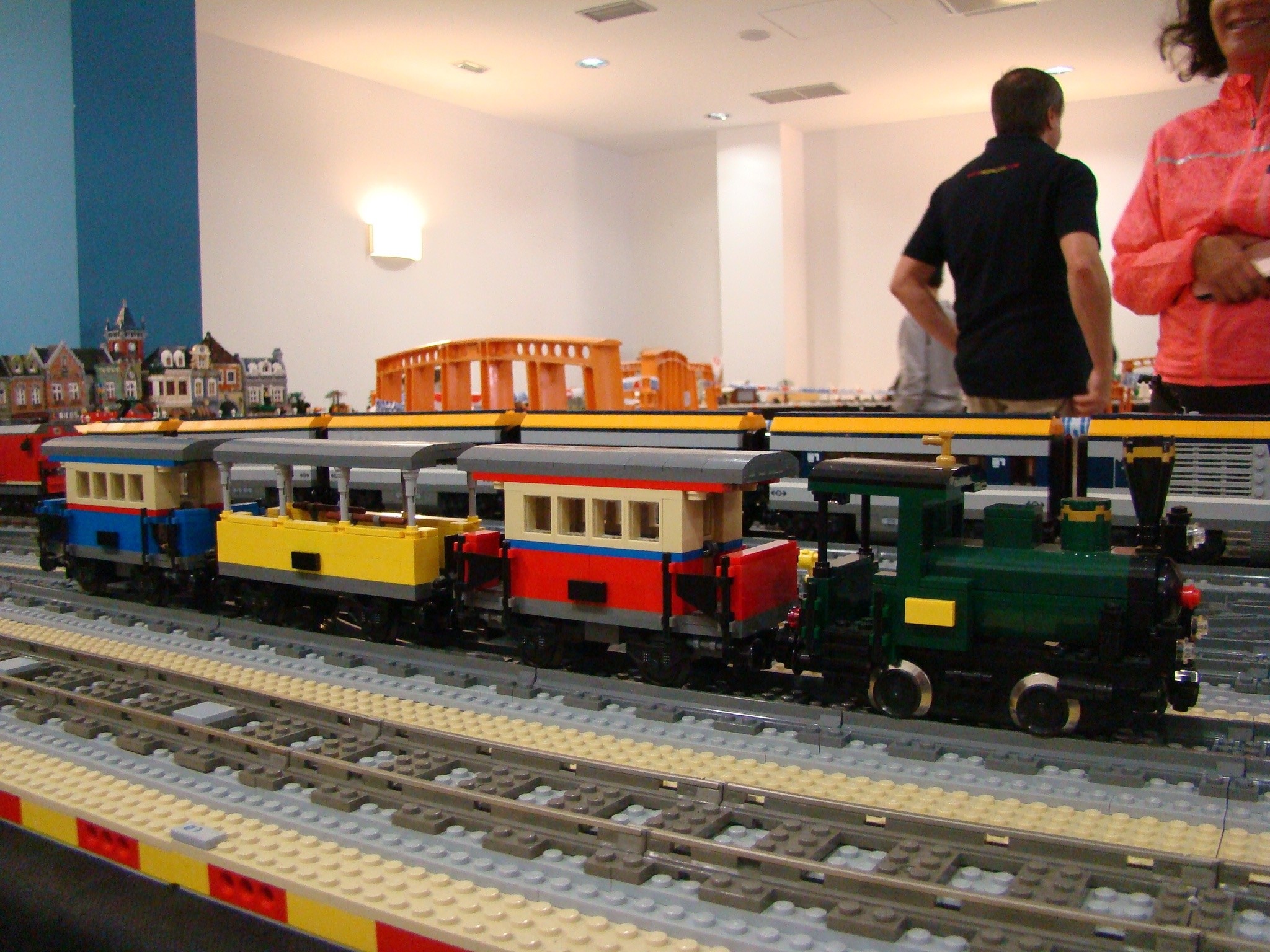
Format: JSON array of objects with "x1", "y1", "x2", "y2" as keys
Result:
[{"x1": 368, "y1": 210, "x2": 422, "y2": 261}]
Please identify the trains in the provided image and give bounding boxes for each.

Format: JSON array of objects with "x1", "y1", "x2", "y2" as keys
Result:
[{"x1": 38, "y1": 412, "x2": 1209, "y2": 737}]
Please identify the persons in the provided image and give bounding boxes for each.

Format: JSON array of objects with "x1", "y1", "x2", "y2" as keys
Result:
[
  {"x1": 1112, "y1": 0, "x2": 1270, "y2": 413},
  {"x1": 889, "y1": 261, "x2": 967, "y2": 416},
  {"x1": 890, "y1": 68, "x2": 1118, "y2": 416}
]
